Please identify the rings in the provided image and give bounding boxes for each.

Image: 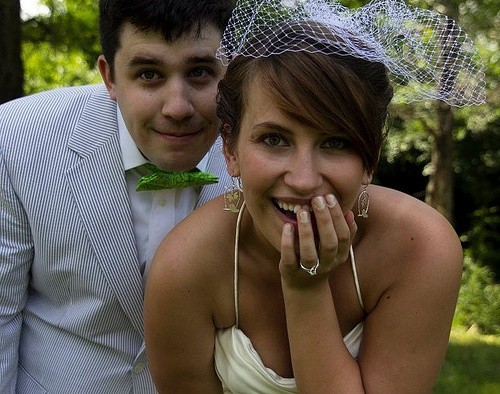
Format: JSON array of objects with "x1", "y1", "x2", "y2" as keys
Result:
[{"x1": 300, "y1": 259, "x2": 321, "y2": 276}]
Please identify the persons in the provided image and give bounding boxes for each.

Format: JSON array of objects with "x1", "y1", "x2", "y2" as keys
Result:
[
  {"x1": 139, "y1": 18, "x2": 466, "y2": 394},
  {"x1": 0, "y1": 0, "x2": 241, "y2": 393}
]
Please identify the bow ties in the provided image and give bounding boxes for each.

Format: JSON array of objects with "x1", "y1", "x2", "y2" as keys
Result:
[{"x1": 134, "y1": 162, "x2": 219, "y2": 191}]
